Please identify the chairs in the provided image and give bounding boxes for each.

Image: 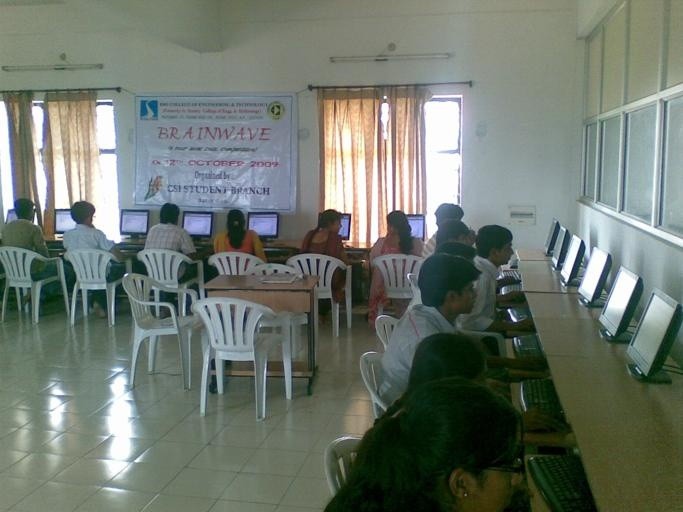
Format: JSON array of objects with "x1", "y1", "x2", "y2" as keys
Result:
[
  {"x1": 324, "y1": 254, "x2": 422, "y2": 504},
  {"x1": 189, "y1": 298, "x2": 302, "y2": 421},
  {"x1": 117, "y1": 270, "x2": 198, "y2": 393}
]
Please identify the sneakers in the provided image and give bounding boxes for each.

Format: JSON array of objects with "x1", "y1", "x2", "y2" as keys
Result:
[{"x1": 93, "y1": 302, "x2": 107, "y2": 318}]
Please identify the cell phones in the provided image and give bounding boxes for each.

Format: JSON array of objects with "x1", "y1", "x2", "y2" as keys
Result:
[{"x1": 535, "y1": 445, "x2": 569, "y2": 456}]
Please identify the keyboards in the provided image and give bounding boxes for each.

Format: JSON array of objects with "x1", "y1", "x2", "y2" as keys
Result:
[
  {"x1": 499, "y1": 282, "x2": 521, "y2": 294},
  {"x1": 520, "y1": 378, "x2": 564, "y2": 418},
  {"x1": 513, "y1": 334, "x2": 544, "y2": 359},
  {"x1": 507, "y1": 305, "x2": 530, "y2": 323},
  {"x1": 116, "y1": 243, "x2": 144, "y2": 251},
  {"x1": 528, "y1": 452, "x2": 597, "y2": 511},
  {"x1": 500, "y1": 269, "x2": 521, "y2": 280},
  {"x1": 45, "y1": 239, "x2": 63, "y2": 248}
]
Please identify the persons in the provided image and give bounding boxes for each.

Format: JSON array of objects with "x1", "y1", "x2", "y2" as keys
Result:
[
  {"x1": 146, "y1": 203, "x2": 202, "y2": 314},
  {"x1": 367, "y1": 210, "x2": 423, "y2": 330},
  {"x1": 1, "y1": 199, "x2": 70, "y2": 304},
  {"x1": 378, "y1": 252, "x2": 572, "y2": 436},
  {"x1": 325, "y1": 377, "x2": 525, "y2": 512},
  {"x1": 435, "y1": 221, "x2": 520, "y2": 293},
  {"x1": 213, "y1": 207, "x2": 266, "y2": 276},
  {"x1": 436, "y1": 242, "x2": 548, "y2": 370},
  {"x1": 423, "y1": 203, "x2": 477, "y2": 257},
  {"x1": 375, "y1": 334, "x2": 533, "y2": 498},
  {"x1": 61, "y1": 202, "x2": 127, "y2": 319},
  {"x1": 459, "y1": 224, "x2": 535, "y2": 357},
  {"x1": 300, "y1": 209, "x2": 350, "y2": 325}
]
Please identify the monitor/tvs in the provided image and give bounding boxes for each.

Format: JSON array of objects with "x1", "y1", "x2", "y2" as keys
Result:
[
  {"x1": 402, "y1": 213, "x2": 425, "y2": 242},
  {"x1": 597, "y1": 265, "x2": 645, "y2": 344},
  {"x1": 622, "y1": 287, "x2": 682, "y2": 385},
  {"x1": 549, "y1": 226, "x2": 570, "y2": 272},
  {"x1": 318, "y1": 211, "x2": 351, "y2": 242},
  {"x1": 120, "y1": 207, "x2": 149, "y2": 240},
  {"x1": 53, "y1": 208, "x2": 78, "y2": 238},
  {"x1": 247, "y1": 211, "x2": 279, "y2": 243},
  {"x1": 182, "y1": 210, "x2": 213, "y2": 243},
  {"x1": 542, "y1": 217, "x2": 561, "y2": 256},
  {"x1": 577, "y1": 246, "x2": 613, "y2": 308},
  {"x1": 5, "y1": 207, "x2": 36, "y2": 224},
  {"x1": 560, "y1": 233, "x2": 586, "y2": 287}
]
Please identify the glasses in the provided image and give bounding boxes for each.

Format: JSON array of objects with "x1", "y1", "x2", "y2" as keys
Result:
[
  {"x1": 486, "y1": 459, "x2": 526, "y2": 474},
  {"x1": 92, "y1": 214, "x2": 97, "y2": 219},
  {"x1": 462, "y1": 287, "x2": 477, "y2": 295}
]
